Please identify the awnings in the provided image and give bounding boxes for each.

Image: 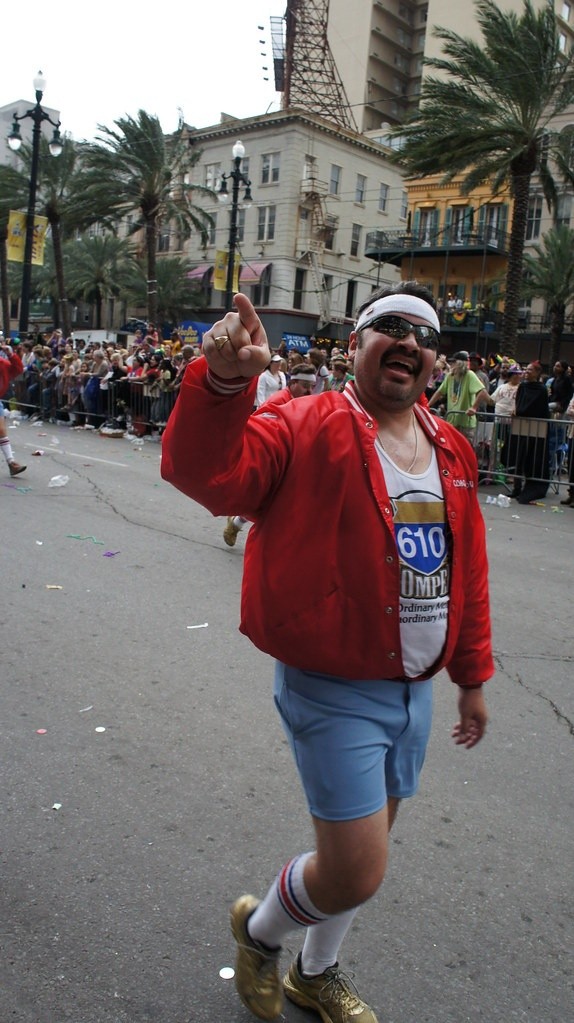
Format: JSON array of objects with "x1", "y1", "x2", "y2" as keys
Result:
[{"x1": 182, "y1": 263, "x2": 272, "y2": 285}]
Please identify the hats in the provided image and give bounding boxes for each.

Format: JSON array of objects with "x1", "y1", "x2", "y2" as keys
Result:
[
  {"x1": 272, "y1": 355, "x2": 284, "y2": 362},
  {"x1": 469, "y1": 351, "x2": 483, "y2": 366},
  {"x1": 508, "y1": 364, "x2": 525, "y2": 373},
  {"x1": 331, "y1": 354, "x2": 346, "y2": 364},
  {"x1": 448, "y1": 350, "x2": 469, "y2": 361}
]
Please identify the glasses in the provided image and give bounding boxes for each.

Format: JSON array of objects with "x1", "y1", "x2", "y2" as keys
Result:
[{"x1": 358, "y1": 314, "x2": 441, "y2": 351}]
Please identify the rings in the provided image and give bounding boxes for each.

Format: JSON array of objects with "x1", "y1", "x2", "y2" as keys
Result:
[{"x1": 214, "y1": 336, "x2": 229, "y2": 349}]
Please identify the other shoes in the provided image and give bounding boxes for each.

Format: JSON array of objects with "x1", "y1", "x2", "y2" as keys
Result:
[{"x1": 8, "y1": 462, "x2": 27, "y2": 476}]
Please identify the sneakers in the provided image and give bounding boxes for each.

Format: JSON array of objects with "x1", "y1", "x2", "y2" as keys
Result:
[
  {"x1": 283, "y1": 949, "x2": 379, "y2": 1023},
  {"x1": 224, "y1": 515, "x2": 242, "y2": 546},
  {"x1": 229, "y1": 893, "x2": 284, "y2": 1023}
]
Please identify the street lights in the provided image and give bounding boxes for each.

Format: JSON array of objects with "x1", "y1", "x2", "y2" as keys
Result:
[
  {"x1": 217, "y1": 138, "x2": 257, "y2": 316},
  {"x1": 8, "y1": 68, "x2": 72, "y2": 342}
]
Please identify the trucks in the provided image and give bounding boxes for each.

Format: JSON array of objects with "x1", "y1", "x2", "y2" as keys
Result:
[{"x1": 72, "y1": 329, "x2": 138, "y2": 352}]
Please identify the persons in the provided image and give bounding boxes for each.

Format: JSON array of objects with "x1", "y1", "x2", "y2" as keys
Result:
[
  {"x1": 160, "y1": 285, "x2": 497, "y2": 1022},
  {"x1": 0, "y1": 333, "x2": 27, "y2": 477},
  {"x1": 223, "y1": 515, "x2": 248, "y2": 545},
  {"x1": 0, "y1": 324, "x2": 573, "y2": 505},
  {"x1": 448, "y1": 294, "x2": 485, "y2": 314}
]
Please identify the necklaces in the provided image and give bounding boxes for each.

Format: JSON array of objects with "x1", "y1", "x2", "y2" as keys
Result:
[{"x1": 376, "y1": 410, "x2": 419, "y2": 472}]
